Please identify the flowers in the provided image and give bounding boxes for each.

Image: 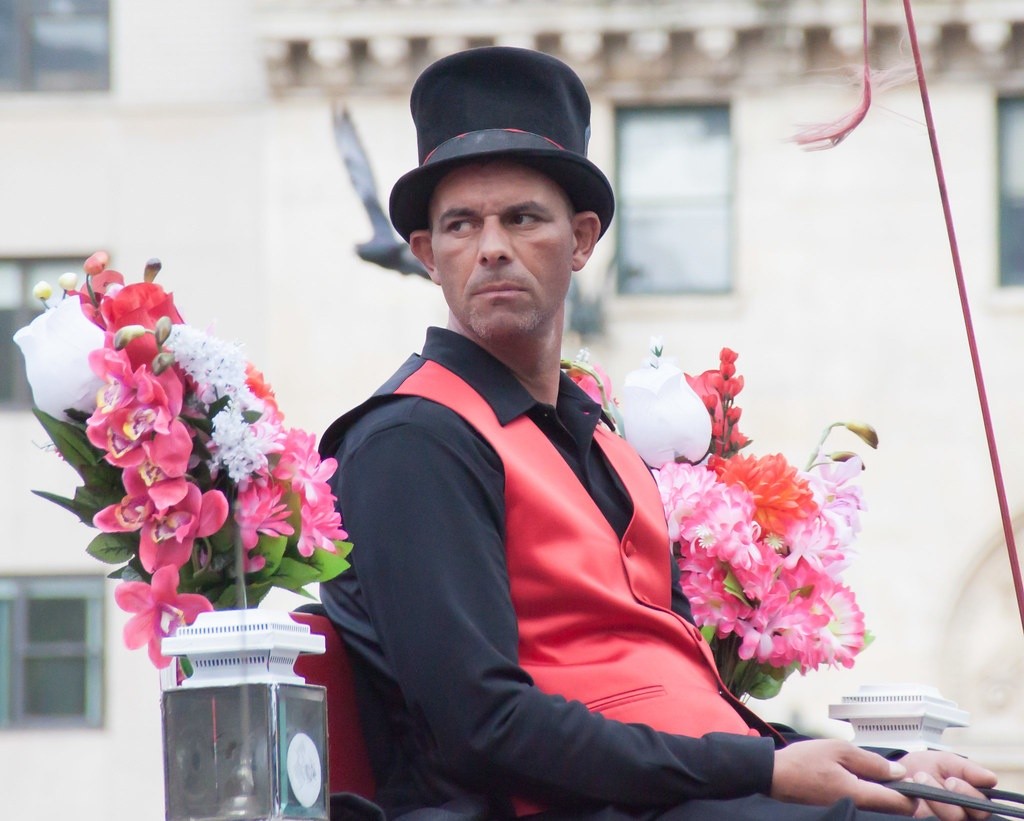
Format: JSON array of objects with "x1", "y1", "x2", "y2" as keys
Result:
[
  {"x1": 14, "y1": 248, "x2": 354, "y2": 680},
  {"x1": 561, "y1": 334, "x2": 880, "y2": 700}
]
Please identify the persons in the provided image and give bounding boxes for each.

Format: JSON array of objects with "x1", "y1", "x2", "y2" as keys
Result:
[{"x1": 312, "y1": 46, "x2": 1000, "y2": 821}]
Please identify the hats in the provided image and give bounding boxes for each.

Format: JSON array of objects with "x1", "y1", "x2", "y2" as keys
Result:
[{"x1": 388, "y1": 47, "x2": 615, "y2": 244}]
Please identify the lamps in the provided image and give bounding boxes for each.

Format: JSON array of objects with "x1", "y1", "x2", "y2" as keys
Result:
[
  {"x1": 160, "y1": 608, "x2": 331, "y2": 821},
  {"x1": 827, "y1": 675, "x2": 972, "y2": 758}
]
